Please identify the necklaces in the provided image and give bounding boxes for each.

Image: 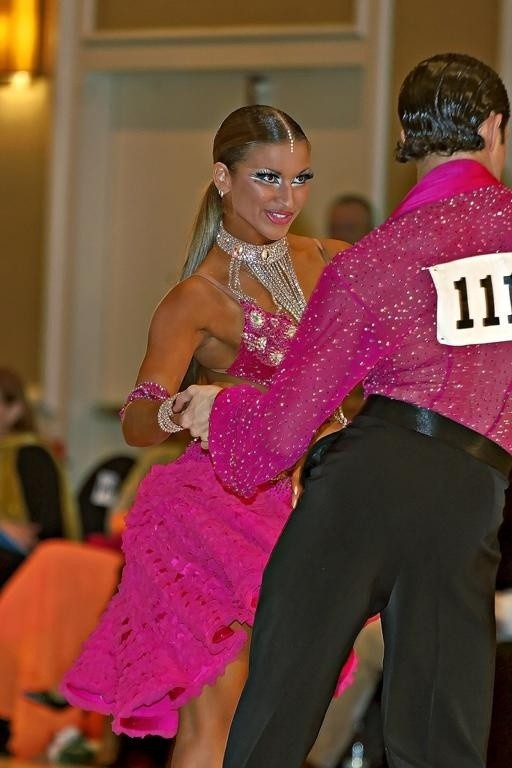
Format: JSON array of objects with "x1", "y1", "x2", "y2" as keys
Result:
[{"x1": 215, "y1": 219, "x2": 308, "y2": 322}]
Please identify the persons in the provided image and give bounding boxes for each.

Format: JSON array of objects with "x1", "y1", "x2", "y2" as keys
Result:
[
  {"x1": 327, "y1": 194, "x2": 374, "y2": 246},
  {"x1": 60, "y1": 99, "x2": 381, "y2": 767},
  {"x1": 170, "y1": 52, "x2": 512, "y2": 764},
  {"x1": 303, "y1": 578, "x2": 512, "y2": 765},
  {"x1": 1, "y1": 363, "x2": 87, "y2": 588}
]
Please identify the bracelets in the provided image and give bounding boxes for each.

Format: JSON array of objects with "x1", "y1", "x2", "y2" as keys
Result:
[{"x1": 158, "y1": 393, "x2": 186, "y2": 435}]
